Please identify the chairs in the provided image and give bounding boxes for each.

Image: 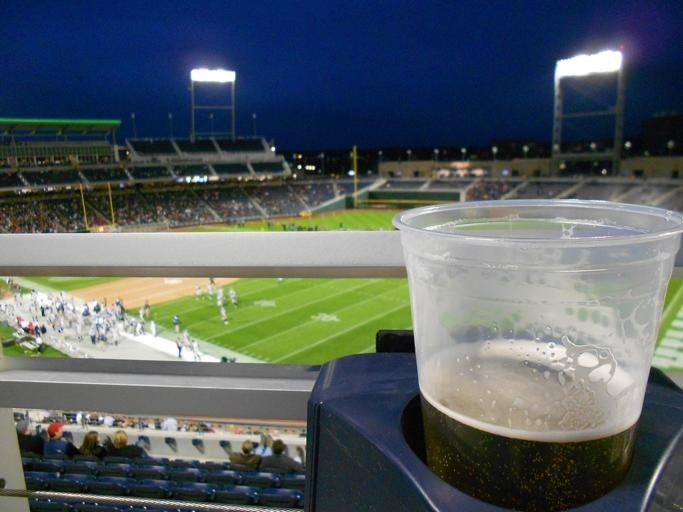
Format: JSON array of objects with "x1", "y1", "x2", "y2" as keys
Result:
[{"x1": 20, "y1": 451, "x2": 306, "y2": 512}]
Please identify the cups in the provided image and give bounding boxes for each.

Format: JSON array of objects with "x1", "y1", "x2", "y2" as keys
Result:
[{"x1": 391, "y1": 197, "x2": 683, "y2": 512}]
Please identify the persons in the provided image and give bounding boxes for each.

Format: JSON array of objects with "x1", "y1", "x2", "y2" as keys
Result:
[
  {"x1": 11, "y1": 407, "x2": 307, "y2": 474},
  {"x1": 466, "y1": 184, "x2": 509, "y2": 200},
  {"x1": 0, "y1": 157, "x2": 344, "y2": 229},
  {"x1": 0, "y1": 275, "x2": 241, "y2": 363}
]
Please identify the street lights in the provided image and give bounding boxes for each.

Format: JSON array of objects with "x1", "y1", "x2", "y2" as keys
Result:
[
  {"x1": 190, "y1": 66, "x2": 237, "y2": 143},
  {"x1": 379, "y1": 144, "x2": 529, "y2": 176},
  {"x1": 549, "y1": 48, "x2": 623, "y2": 178}
]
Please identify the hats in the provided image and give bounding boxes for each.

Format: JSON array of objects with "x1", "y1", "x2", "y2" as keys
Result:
[{"x1": 48, "y1": 423, "x2": 63, "y2": 436}]
